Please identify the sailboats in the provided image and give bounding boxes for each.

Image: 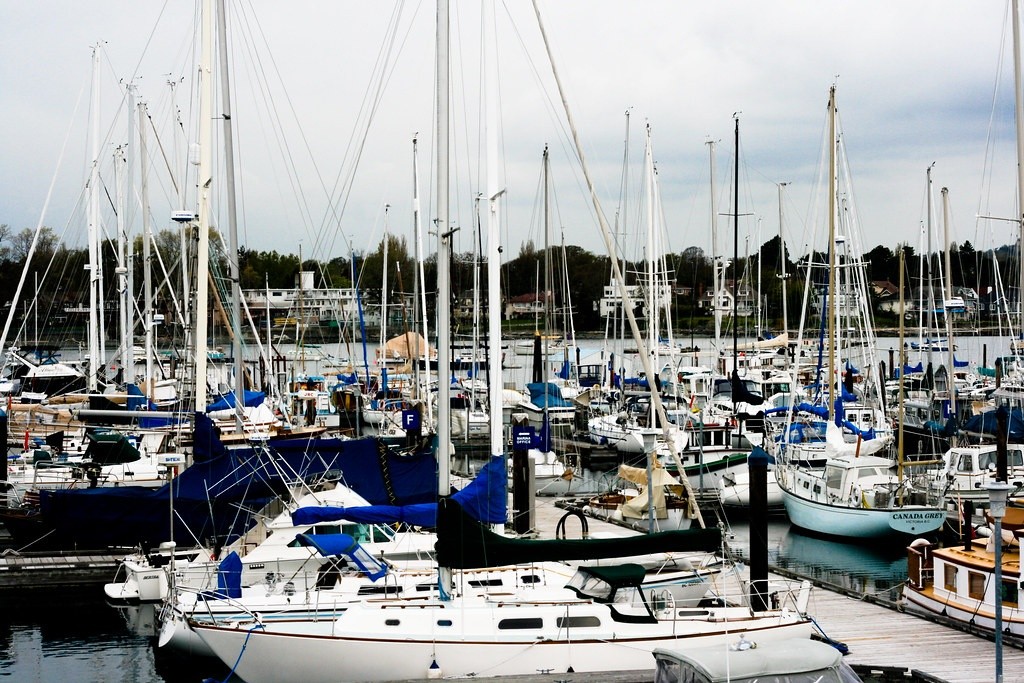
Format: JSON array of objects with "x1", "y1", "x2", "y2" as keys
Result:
[{"x1": 0, "y1": 0, "x2": 1024, "y2": 683}]
